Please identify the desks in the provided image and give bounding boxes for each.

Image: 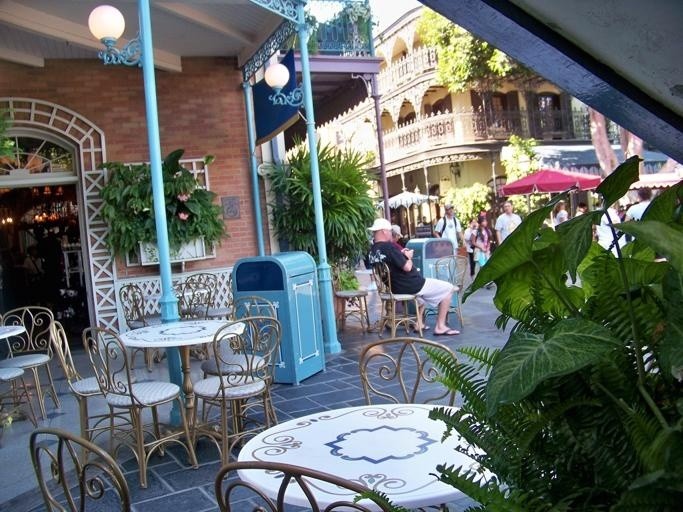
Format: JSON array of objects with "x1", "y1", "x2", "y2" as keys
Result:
[{"x1": 236, "y1": 403, "x2": 500, "y2": 512}]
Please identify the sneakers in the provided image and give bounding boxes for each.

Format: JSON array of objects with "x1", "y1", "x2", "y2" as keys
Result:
[{"x1": 486, "y1": 282, "x2": 495, "y2": 288}]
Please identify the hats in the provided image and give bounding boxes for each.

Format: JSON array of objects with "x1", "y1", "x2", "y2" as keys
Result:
[
  {"x1": 445, "y1": 204, "x2": 453, "y2": 210},
  {"x1": 367, "y1": 218, "x2": 392, "y2": 231},
  {"x1": 391, "y1": 225, "x2": 403, "y2": 238}
]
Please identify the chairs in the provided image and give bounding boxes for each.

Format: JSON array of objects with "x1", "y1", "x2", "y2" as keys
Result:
[
  {"x1": 371, "y1": 262, "x2": 424, "y2": 338},
  {"x1": 330, "y1": 264, "x2": 371, "y2": 333},
  {"x1": 360, "y1": 336, "x2": 459, "y2": 407},
  {"x1": 425, "y1": 255, "x2": 467, "y2": 328}
]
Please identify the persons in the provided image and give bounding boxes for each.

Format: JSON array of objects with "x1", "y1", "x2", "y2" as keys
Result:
[
  {"x1": 553, "y1": 201, "x2": 568, "y2": 223},
  {"x1": 471, "y1": 216, "x2": 496, "y2": 289},
  {"x1": 423, "y1": 216, "x2": 431, "y2": 225},
  {"x1": 419, "y1": 216, "x2": 423, "y2": 227},
  {"x1": 465, "y1": 218, "x2": 478, "y2": 281},
  {"x1": 386, "y1": 225, "x2": 411, "y2": 329},
  {"x1": 601, "y1": 203, "x2": 621, "y2": 229},
  {"x1": 625, "y1": 187, "x2": 653, "y2": 241},
  {"x1": 366, "y1": 218, "x2": 459, "y2": 336},
  {"x1": 20, "y1": 245, "x2": 46, "y2": 306},
  {"x1": 434, "y1": 203, "x2": 467, "y2": 292},
  {"x1": 494, "y1": 202, "x2": 522, "y2": 245},
  {"x1": 575, "y1": 202, "x2": 587, "y2": 218}
]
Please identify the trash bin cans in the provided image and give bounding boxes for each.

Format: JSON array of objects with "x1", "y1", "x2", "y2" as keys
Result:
[
  {"x1": 404, "y1": 239, "x2": 459, "y2": 314},
  {"x1": 232, "y1": 252, "x2": 326, "y2": 386}
]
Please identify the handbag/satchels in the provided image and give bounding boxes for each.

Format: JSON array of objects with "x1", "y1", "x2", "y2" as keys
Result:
[
  {"x1": 397, "y1": 234, "x2": 409, "y2": 247},
  {"x1": 479, "y1": 250, "x2": 487, "y2": 267},
  {"x1": 469, "y1": 230, "x2": 478, "y2": 249}
]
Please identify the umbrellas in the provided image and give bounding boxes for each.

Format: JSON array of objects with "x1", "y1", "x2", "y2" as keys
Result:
[
  {"x1": 495, "y1": 162, "x2": 604, "y2": 200},
  {"x1": 379, "y1": 191, "x2": 438, "y2": 239}
]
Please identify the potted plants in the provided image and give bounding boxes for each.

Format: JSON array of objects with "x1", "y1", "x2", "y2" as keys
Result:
[{"x1": 255, "y1": 135, "x2": 384, "y2": 332}]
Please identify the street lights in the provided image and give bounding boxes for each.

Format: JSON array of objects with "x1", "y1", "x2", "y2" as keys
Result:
[
  {"x1": 87, "y1": 0, "x2": 189, "y2": 427},
  {"x1": 262, "y1": 31, "x2": 341, "y2": 353}
]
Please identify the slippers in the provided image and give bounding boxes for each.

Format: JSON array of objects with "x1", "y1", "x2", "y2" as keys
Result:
[
  {"x1": 414, "y1": 324, "x2": 430, "y2": 333},
  {"x1": 433, "y1": 327, "x2": 460, "y2": 336}
]
